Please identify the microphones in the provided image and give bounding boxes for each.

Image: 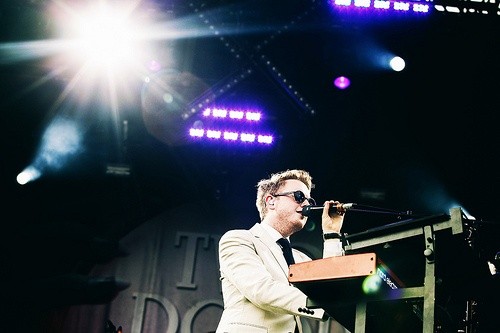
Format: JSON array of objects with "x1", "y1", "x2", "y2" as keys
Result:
[{"x1": 302, "y1": 203, "x2": 357, "y2": 216}]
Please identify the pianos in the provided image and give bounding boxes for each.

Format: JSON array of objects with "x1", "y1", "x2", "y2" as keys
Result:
[
  {"x1": 289, "y1": 253, "x2": 437, "y2": 333},
  {"x1": 343, "y1": 207, "x2": 500, "y2": 333}
]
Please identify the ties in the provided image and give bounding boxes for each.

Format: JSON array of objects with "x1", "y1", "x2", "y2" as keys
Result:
[{"x1": 276, "y1": 238, "x2": 295, "y2": 267}]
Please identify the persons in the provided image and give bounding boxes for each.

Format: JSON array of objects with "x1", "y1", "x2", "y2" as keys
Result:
[{"x1": 214, "y1": 167, "x2": 346, "y2": 333}]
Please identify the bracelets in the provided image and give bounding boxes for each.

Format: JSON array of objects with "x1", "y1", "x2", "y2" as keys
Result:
[{"x1": 322, "y1": 232, "x2": 343, "y2": 241}]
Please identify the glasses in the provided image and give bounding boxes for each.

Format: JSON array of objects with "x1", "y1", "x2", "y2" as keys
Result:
[{"x1": 273, "y1": 190, "x2": 316, "y2": 207}]
